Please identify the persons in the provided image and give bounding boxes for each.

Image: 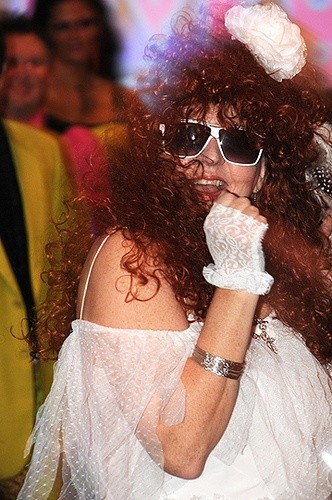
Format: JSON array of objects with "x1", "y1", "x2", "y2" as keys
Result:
[
  {"x1": 36, "y1": 37, "x2": 332, "y2": 500},
  {"x1": 25, "y1": 0, "x2": 158, "y2": 170},
  {"x1": 0, "y1": 8, "x2": 111, "y2": 220},
  {"x1": 4, "y1": 117, "x2": 71, "y2": 495}
]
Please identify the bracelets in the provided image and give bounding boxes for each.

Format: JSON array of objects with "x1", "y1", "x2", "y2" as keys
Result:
[{"x1": 190, "y1": 343, "x2": 246, "y2": 381}]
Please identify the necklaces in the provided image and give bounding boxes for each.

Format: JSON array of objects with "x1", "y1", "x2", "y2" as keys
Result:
[{"x1": 237, "y1": 314, "x2": 281, "y2": 355}]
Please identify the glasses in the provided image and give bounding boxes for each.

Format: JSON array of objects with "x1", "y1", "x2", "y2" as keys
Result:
[{"x1": 159, "y1": 118, "x2": 266, "y2": 166}]
